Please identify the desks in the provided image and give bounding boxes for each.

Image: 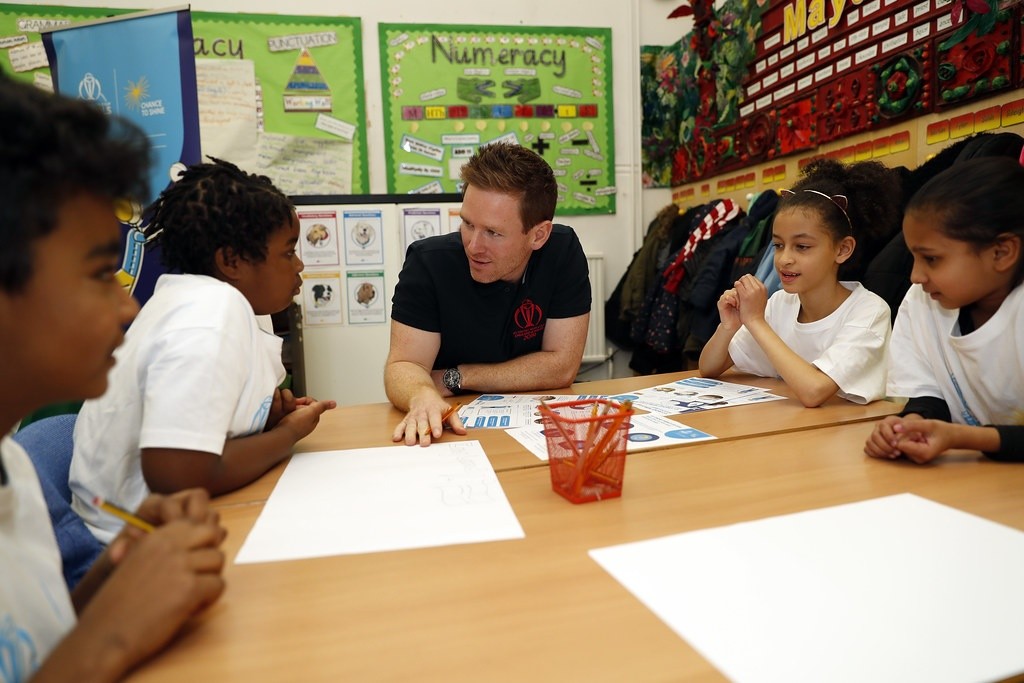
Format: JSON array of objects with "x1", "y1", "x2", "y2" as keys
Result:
[{"x1": 115, "y1": 365, "x2": 1024, "y2": 683}]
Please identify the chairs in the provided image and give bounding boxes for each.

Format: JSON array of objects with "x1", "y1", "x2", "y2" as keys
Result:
[{"x1": 8, "y1": 414, "x2": 105, "y2": 591}]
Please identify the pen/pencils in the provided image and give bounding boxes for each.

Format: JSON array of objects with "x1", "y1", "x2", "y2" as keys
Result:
[
  {"x1": 424, "y1": 401, "x2": 464, "y2": 436},
  {"x1": 92, "y1": 497, "x2": 155, "y2": 536},
  {"x1": 539, "y1": 396, "x2": 633, "y2": 503}
]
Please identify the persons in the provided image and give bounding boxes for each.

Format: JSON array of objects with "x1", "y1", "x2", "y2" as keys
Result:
[
  {"x1": 383, "y1": 142, "x2": 592, "y2": 444},
  {"x1": 68, "y1": 157, "x2": 337, "y2": 545},
  {"x1": 0, "y1": 71, "x2": 229, "y2": 683},
  {"x1": 864, "y1": 131, "x2": 1024, "y2": 465},
  {"x1": 699, "y1": 157, "x2": 892, "y2": 408}
]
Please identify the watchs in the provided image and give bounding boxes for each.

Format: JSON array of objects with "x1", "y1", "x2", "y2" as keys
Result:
[{"x1": 443, "y1": 365, "x2": 463, "y2": 396}]
